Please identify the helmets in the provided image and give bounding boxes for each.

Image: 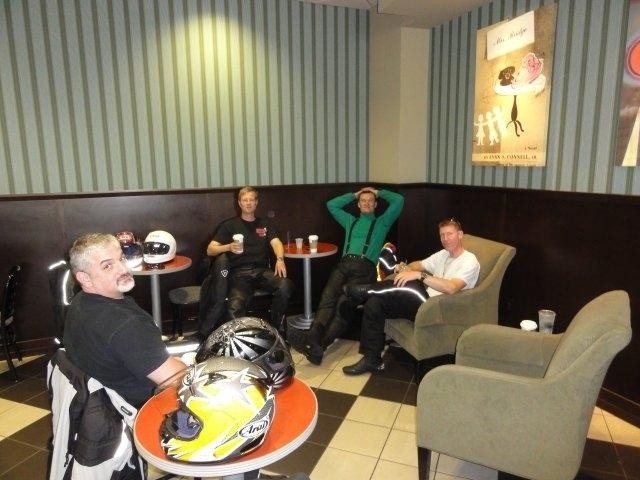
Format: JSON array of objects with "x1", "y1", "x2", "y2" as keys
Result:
[
  {"x1": 143, "y1": 230, "x2": 177, "y2": 265},
  {"x1": 120, "y1": 241, "x2": 144, "y2": 268},
  {"x1": 194, "y1": 317, "x2": 296, "y2": 391},
  {"x1": 159, "y1": 357, "x2": 276, "y2": 464}
]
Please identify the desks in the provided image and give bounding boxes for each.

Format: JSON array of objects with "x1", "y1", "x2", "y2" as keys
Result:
[
  {"x1": 281, "y1": 242, "x2": 337, "y2": 330},
  {"x1": 131, "y1": 374, "x2": 320, "y2": 479},
  {"x1": 125, "y1": 255, "x2": 192, "y2": 334}
]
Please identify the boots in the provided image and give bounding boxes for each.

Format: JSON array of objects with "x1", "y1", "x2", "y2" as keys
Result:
[{"x1": 287, "y1": 320, "x2": 335, "y2": 364}]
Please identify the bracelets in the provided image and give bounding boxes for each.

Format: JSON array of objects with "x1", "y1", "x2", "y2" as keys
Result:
[
  {"x1": 419, "y1": 271, "x2": 429, "y2": 285},
  {"x1": 277, "y1": 257, "x2": 285, "y2": 262}
]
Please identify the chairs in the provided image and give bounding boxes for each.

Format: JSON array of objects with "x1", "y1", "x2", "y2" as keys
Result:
[
  {"x1": 169, "y1": 258, "x2": 214, "y2": 337},
  {"x1": 0, "y1": 263, "x2": 25, "y2": 383}
]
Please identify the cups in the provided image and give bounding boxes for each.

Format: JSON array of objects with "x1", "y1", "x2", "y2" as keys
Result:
[
  {"x1": 296, "y1": 239, "x2": 303, "y2": 248},
  {"x1": 307, "y1": 235, "x2": 319, "y2": 252},
  {"x1": 233, "y1": 233, "x2": 244, "y2": 255},
  {"x1": 538, "y1": 310, "x2": 556, "y2": 334},
  {"x1": 521, "y1": 320, "x2": 536, "y2": 332}
]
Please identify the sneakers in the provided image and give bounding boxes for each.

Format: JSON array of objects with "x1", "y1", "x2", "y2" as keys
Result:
[
  {"x1": 343, "y1": 356, "x2": 382, "y2": 374},
  {"x1": 343, "y1": 285, "x2": 367, "y2": 302}
]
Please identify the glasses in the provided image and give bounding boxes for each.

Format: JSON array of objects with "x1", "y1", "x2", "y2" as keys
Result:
[
  {"x1": 145, "y1": 264, "x2": 165, "y2": 269},
  {"x1": 448, "y1": 216, "x2": 460, "y2": 229}
]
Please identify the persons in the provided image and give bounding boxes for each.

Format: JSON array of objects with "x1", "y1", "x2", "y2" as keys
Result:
[
  {"x1": 340, "y1": 216, "x2": 481, "y2": 376},
  {"x1": 206, "y1": 187, "x2": 296, "y2": 351},
  {"x1": 287, "y1": 185, "x2": 406, "y2": 367},
  {"x1": 59, "y1": 230, "x2": 193, "y2": 409}
]
